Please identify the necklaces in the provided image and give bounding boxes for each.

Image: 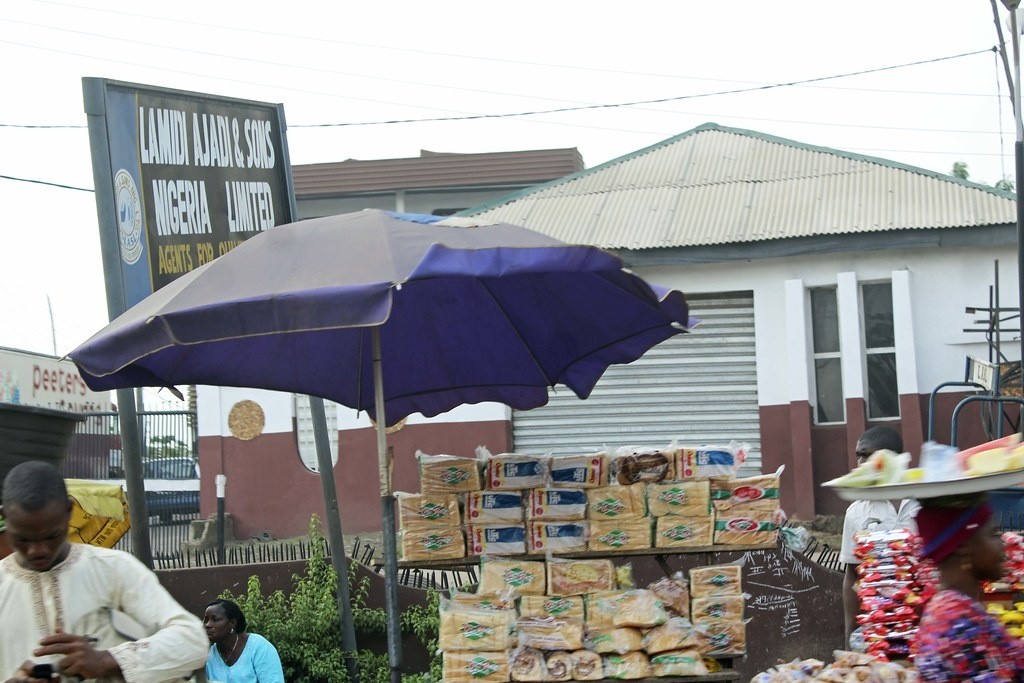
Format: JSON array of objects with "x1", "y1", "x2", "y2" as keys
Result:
[{"x1": 218, "y1": 634, "x2": 239, "y2": 664}]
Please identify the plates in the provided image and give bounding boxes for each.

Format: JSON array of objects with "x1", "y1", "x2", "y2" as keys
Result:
[{"x1": 821, "y1": 469, "x2": 1024, "y2": 500}]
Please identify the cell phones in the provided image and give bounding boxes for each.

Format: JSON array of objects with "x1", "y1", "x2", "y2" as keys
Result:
[{"x1": 32, "y1": 665, "x2": 52, "y2": 683}]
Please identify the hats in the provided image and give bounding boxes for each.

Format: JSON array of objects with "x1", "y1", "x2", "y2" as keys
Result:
[{"x1": 913, "y1": 497, "x2": 994, "y2": 565}]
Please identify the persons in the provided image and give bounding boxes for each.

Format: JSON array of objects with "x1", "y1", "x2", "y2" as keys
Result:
[
  {"x1": 1, "y1": 459, "x2": 212, "y2": 683},
  {"x1": 201, "y1": 598, "x2": 286, "y2": 683},
  {"x1": 837, "y1": 427, "x2": 920, "y2": 657},
  {"x1": 913, "y1": 491, "x2": 1023, "y2": 683}
]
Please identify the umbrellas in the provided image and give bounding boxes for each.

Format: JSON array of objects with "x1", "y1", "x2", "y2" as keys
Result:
[
  {"x1": 55, "y1": 207, "x2": 701, "y2": 683},
  {"x1": 58, "y1": 479, "x2": 134, "y2": 551}
]
